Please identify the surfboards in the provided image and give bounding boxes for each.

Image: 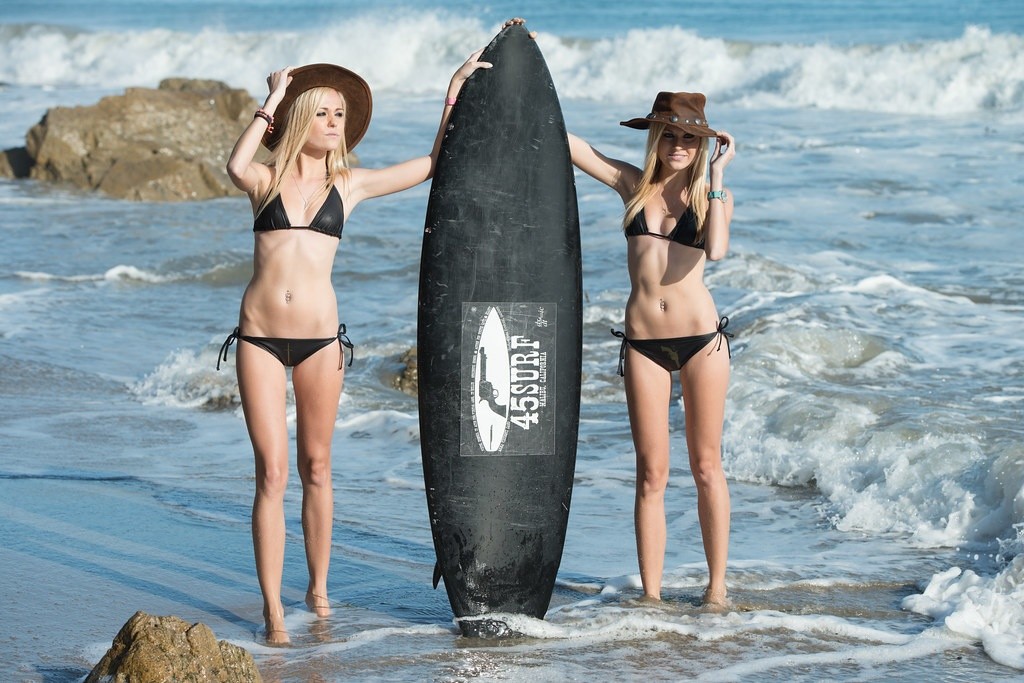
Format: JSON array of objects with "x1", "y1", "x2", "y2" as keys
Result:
[{"x1": 414, "y1": 20, "x2": 587, "y2": 644}]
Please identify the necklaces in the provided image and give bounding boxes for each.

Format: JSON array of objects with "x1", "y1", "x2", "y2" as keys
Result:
[{"x1": 291, "y1": 171, "x2": 328, "y2": 209}]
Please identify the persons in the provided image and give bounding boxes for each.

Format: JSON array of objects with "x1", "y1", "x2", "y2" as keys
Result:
[
  {"x1": 502, "y1": 17, "x2": 736, "y2": 613},
  {"x1": 226, "y1": 46, "x2": 494, "y2": 648}
]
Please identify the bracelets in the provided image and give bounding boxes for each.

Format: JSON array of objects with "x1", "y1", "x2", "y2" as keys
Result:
[
  {"x1": 445, "y1": 98, "x2": 457, "y2": 105},
  {"x1": 254, "y1": 109, "x2": 275, "y2": 134}
]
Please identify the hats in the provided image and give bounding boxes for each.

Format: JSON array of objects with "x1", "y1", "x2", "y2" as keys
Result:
[
  {"x1": 261, "y1": 63, "x2": 373, "y2": 158},
  {"x1": 620, "y1": 91, "x2": 727, "y2": 146}
]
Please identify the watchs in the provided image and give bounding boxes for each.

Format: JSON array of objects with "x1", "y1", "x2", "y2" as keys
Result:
[{"x1": 707, "y1": 189, "x2": 728, "y2": 203}]
